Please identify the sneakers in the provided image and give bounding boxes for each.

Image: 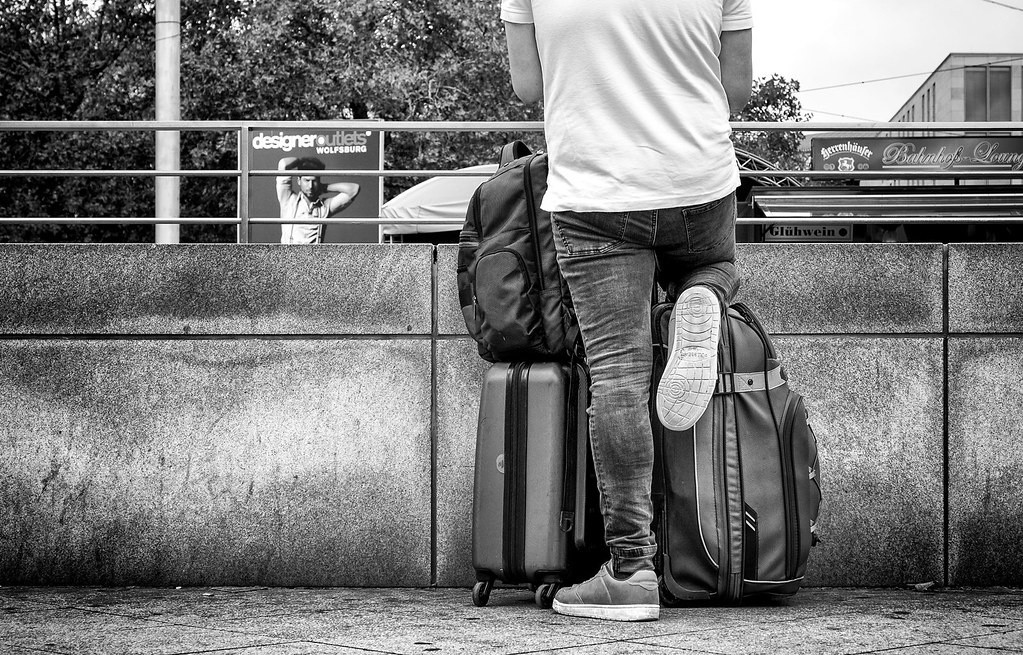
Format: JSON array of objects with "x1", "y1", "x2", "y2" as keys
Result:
[
  {"x1": 656, "y1": 282, "x2": 724, "y2": 432},
  {"x1": 552, "y1": 556, "x2": 660, "y2": 620}
]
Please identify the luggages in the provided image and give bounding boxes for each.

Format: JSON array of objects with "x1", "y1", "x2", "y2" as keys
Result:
[{"x1": 472, "y1": 360, "x2": 598, "y2": 607}]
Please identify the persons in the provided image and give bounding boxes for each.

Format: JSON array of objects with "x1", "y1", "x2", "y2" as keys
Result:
[
  {"x1": 276, "y1": 157, "x2": 361, "y2": 246},
  {"x1": 500, "y1": 0, "x2": 751, "y2": 621}
]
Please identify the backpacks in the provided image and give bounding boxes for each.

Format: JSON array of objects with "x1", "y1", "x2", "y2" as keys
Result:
[
  {"x1": 649, "y1": 302, "x2": 823, "y2": 606},
  {"x1": 455, "y1": 140, "x2": 587, "y2": 364}
]
